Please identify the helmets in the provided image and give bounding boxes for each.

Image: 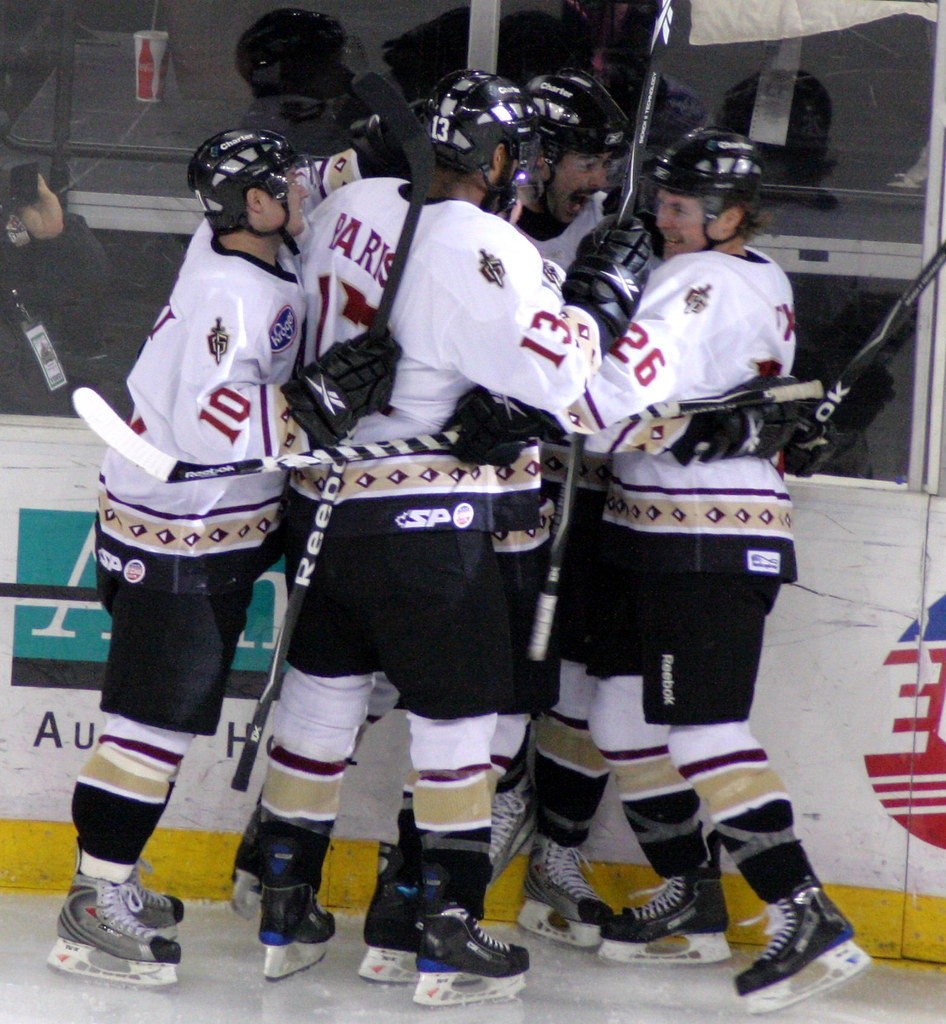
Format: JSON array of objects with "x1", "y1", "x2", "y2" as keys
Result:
[
  {"x1": 524, "y1": 70, "x2": 627, "y2": 159},
  {"x1": 185, "y1": 134, "x2": 300, "y2": 232},
  {"x1": 722, "y1": 71, "x2": 831, "y2": 180},
  {"x1": 234, "y1": 8, "x2": 344, "y2": 99},
  {"x1": 647, "y1": 124, "x2": 764, "y2": 201},
  {"x1": 424, "y1": 73, "x2": 541, "y2": 173}
]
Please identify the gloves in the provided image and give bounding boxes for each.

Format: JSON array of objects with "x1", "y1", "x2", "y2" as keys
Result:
[
  {"x1": 561, "y1": 209, "x2": 649, "y2": 341},
  {"x1": 440, "y1": 382, "x2": 565, "y2": 469},
  {"x1": 787, "y1": 405, "x2": 860, "y2": 476},
  {"x1": 670, "y1": 373, "x2": 805, "y2": 467},
  {"x1": 277, "y1": 326, "x2": 402, "y2": 450}
]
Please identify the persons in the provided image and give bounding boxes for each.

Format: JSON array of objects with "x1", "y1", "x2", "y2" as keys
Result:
[
  {"x1": 256, "y1": 69, "x2": 657, "y2": 1008},
  {"x1": 443, "y1": 128, "x2": 867, "y2": 1013},
  {"x1": 230, "y1": 62, "x2": 607, "y2": 984},
  {"x1": 0, "y1": 172, "x2": 115, "y2": 415},
  {"x1": 46, "y1": 126, "x2": 400, "y2": 984},
  {"x1": 236, "y1": 0, "x2": 897, "y2": 482}
]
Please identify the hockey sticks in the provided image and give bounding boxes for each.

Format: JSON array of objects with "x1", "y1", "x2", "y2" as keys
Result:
[
  {"x1": 69, "y1": 376, "x2": 825, "y2": 487},
  {"x1": 228, "y1": 70, "x2": 440, "y2": 793},
  {"x1": 815, "y1": 241, "x2": 946, "y2": 427},
  {"x1": 526, "y1": 0, "x2": 679, "y2": 666}
]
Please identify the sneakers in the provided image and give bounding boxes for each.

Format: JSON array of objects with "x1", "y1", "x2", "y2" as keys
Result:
[
  {"x1": 730, "y1": 883, "x2": 870, "y2": 1016},
  {"x1": 485, "y1": 770, "x2": 536, "y2": 881},
  {"x1": 74, "y1": 838, "x2": 184, "y2": 943},
  {"x1": 598, "y1": 821, "x2": 730, "y2": 969},
  {"x1": 516, "y1": 828, "x2": 613, "y2": 950},
  {"x1": 357, "y1": 842, "x2": 422, "y2": 986},
  {"x1": 259, "y1": 813, "x2": 335, "y2": 982},
  {"x1": 48, "y1": 855, "x2": 183, "y2": 987},
  {"x1": 229, "y1": 799, "x2": 276, "y2": 919},
  {"x1": 411, "y1": 866, "x2": 531, "y2": 1008}
]
